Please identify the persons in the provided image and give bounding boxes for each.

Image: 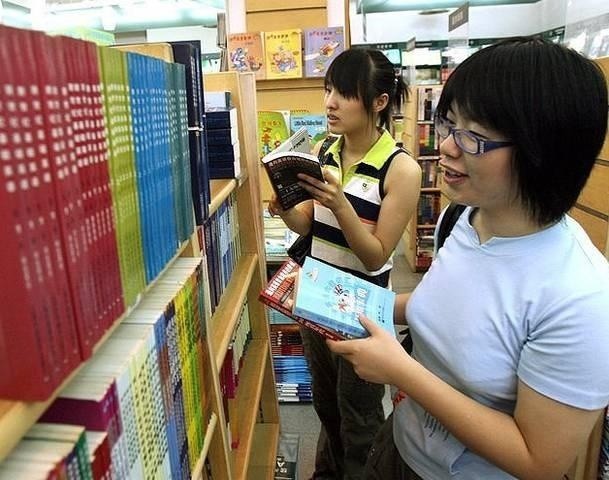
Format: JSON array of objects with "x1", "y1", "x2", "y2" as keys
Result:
[
  {"x1": 266, "y1": 45, "x2": 420, "y2": 480},
  {"x1": 323, "y1": 35, "x2": 607, "y2": 480}
]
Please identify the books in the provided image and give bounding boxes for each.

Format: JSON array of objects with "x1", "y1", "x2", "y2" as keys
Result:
[
  {"x1": 0, "y1": 23, "x2": 253, "y2": 480},
  {"x1": 221, "y1": 26, "x2": 352, "y2": 160},
  {"x1": 258, "y1": 128, "x2": 327, "y2": 207},
  {"x1": 415, "y1": 81, "x2": 454, "y2": 267},
  {"x1": 258, "y1": 256, "x2": 360, "y2": 343},
  {"x1": 256, "y1": 199, "x2": 315, "y2": 406},
  {"x1": 293, "y1": 251, "x2": 403, "y2": 346},
  {"x1": 390, "y1": 92, "x2": 405, "y2": 144},
  {"x1": 273, "y1": 432, "x2": 300, "y2": 480}
]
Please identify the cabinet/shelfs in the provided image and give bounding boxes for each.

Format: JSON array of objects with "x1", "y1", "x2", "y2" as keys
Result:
[{"x1": 1, "y1": 40, "x2": 285, "y2": 480}]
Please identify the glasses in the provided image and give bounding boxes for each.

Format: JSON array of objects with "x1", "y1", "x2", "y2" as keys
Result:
[{"x1": 433, "y1": 115, "x2": 512, "y2": 156}]
